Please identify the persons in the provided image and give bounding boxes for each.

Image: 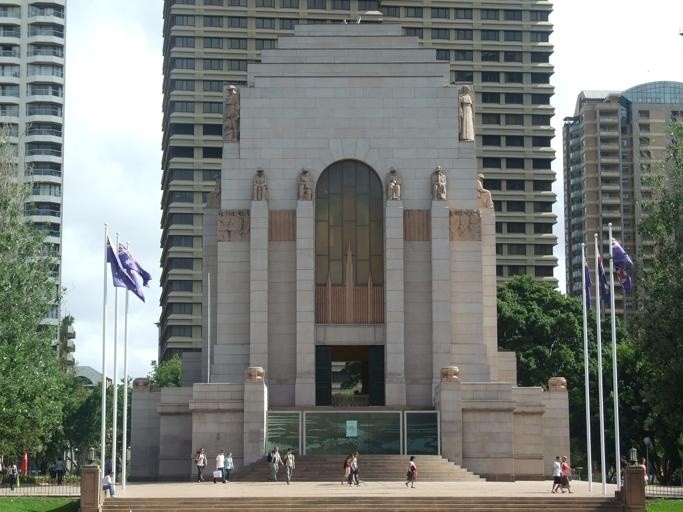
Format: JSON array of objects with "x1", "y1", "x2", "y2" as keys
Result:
[
  {"x1": 224, "y1": 452, "x2": 235, "y2": 483},
  {"x1": 384, "y1": 166, "x2": 402, "y2": 201},
  {"x1": 340, "y1": 453, "x2": 358, "y2": 486},
  {"x1": 475, "y1": 173, "x2": 493, "y2": 208},
  {"x1": 555, "y1": 455, "x2": 575, "y2": 493},
  {"x1": 193, "y1": 448, "x2": 208, "y2": 483},
  {"x1": 266, "y1": 446, "x2": 284, "y2": 482},
  {"x1": 54, "y1": 457, "x2": 65, "y2": 485},
  {"x1": 403, "y1": 455, "x2": 417, "y2": 487},
  {"x1": 295, "y1": 166, "x2": 313, "y2": 201},
  {"x1": 639, "y1": 456, "x2": 648, "y2": 486},
  {"x1": 204, "y1": 174, "x2": 219, "y2": 208},
  {"x1": 551, "y1": 456, "x2": 566, "y2": 493},
  {"x1": 347, "y1": 452, "x2": 360, "y2": 486},
  {"x1": 250, "y1": 167, "x2": 268, "y2": 201},
  {"x1": 458, "y1": 83, "x2": 475, "y2": 142},
  {"x1": 282, "y1": 448, "x2": 296, "y2": 484},
  {"x1": 220, "y1": 83, "x2": 239, "y2": 140},
  {"x1": 213, "y1": 448, "x2": 228, "y2": 483},
  {"x1": 429, "y1": 165, "x2": 446, "y2": 201},
  {"x1": 7, "y1": 460, "x2": 18, "y2": 492},
  {"x1": 102, "y1": 453, "x2": 124, "y2": 496}
]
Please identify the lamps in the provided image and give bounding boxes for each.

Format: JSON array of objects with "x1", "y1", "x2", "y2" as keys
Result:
[
  {"x1": 629, "y1": 448, "x2": 638, "y2": 466},
  {"x1": 87, "y1": 447, "x2": 96, "y2": 464}
]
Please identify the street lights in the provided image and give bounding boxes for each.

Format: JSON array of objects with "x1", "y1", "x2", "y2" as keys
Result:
[{"x1": 643, "y1": 437, "x2": 651, "y2": 484}]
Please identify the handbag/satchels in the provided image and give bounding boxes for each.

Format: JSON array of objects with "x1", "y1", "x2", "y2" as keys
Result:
[{"x1": 213, "y1": 470, "x2": 222, "y2": 478}]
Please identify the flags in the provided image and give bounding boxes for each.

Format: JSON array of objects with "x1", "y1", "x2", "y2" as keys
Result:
[
  {"x1": 597, "y1": 252, "x2": 610, "y2": 305},
  {"x1": 105, "y1": 237, "x2": 147, "y2": 303},
  {"x1": 114, "y1": 240, "x2": 153, "y2": 288},
  {"x1": 611, "y1": 237, "x2": 634, "y2": 293},
  {"x1": 584, "y1": 259, "x2": 592, "y2": 313}
]
